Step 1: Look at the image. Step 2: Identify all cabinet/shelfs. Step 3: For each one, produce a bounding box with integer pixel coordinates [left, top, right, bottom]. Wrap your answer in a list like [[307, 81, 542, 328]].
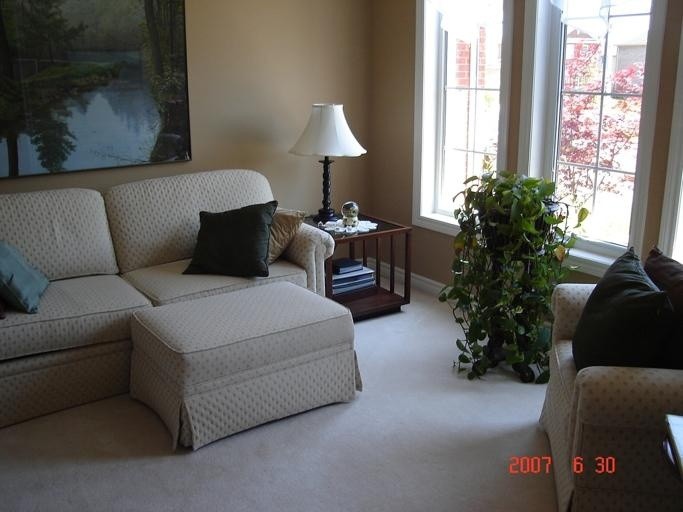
[[304, 211, 412, 321]]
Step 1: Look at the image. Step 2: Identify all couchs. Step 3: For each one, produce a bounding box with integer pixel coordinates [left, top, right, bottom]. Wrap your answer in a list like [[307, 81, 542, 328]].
[[1, 170, 335, 424]]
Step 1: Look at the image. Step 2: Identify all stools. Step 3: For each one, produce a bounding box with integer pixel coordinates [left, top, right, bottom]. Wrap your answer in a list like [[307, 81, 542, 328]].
[[129, 279, 362, 451]]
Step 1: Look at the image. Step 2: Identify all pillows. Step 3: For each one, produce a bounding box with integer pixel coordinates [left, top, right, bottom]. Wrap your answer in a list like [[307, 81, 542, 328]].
[[1, 239, 51, 313], [181, 201, 306, 277], [571, 245, 683, 372]]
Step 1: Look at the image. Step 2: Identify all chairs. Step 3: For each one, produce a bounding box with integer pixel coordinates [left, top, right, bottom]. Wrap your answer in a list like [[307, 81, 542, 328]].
[[537, 283, 682, 511]]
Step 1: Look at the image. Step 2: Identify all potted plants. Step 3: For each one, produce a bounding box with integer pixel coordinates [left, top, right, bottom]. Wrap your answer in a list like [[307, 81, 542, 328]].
[[438, 169, 588, 383]]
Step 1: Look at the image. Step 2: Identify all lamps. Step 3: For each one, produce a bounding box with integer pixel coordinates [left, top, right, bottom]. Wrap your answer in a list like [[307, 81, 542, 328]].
[[288, 103, 367, 224]]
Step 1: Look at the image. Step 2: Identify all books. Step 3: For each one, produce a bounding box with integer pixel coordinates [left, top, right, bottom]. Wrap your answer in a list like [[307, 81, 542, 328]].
[[328, 257, 378, 297]]
